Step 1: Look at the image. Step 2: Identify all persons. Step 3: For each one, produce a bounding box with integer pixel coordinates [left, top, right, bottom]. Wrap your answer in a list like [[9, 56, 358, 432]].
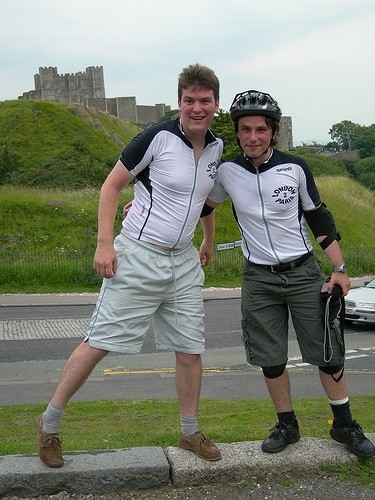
[[36, 62, 224, 468], [123, 90, 375, 459]]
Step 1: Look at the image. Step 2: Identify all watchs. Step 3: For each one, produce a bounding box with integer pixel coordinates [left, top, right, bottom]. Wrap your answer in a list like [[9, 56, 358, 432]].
[[333, 263, 348, 273]]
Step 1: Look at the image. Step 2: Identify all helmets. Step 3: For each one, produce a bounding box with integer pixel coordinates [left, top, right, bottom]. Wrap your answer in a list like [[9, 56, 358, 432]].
[[230, 90, 282, 122]]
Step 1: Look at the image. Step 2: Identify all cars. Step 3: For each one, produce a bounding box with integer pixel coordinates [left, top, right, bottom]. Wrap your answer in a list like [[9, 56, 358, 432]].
[[344, 277, 375, 328]]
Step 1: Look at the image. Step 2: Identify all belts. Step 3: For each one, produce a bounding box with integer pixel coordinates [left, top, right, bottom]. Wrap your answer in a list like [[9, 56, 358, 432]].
[[243, 250, 314, 273]]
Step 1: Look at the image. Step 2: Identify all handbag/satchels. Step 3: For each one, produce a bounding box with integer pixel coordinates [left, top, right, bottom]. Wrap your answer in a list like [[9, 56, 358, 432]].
[[320, 277, 346, 382]]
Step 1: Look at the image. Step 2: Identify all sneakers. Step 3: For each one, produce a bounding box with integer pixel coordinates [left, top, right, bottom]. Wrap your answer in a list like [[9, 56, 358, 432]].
[[261, 420, 300, 453], [179, 430, 221, 461], [36, 414, 64, 468], [330, 419, 375, 459]]
